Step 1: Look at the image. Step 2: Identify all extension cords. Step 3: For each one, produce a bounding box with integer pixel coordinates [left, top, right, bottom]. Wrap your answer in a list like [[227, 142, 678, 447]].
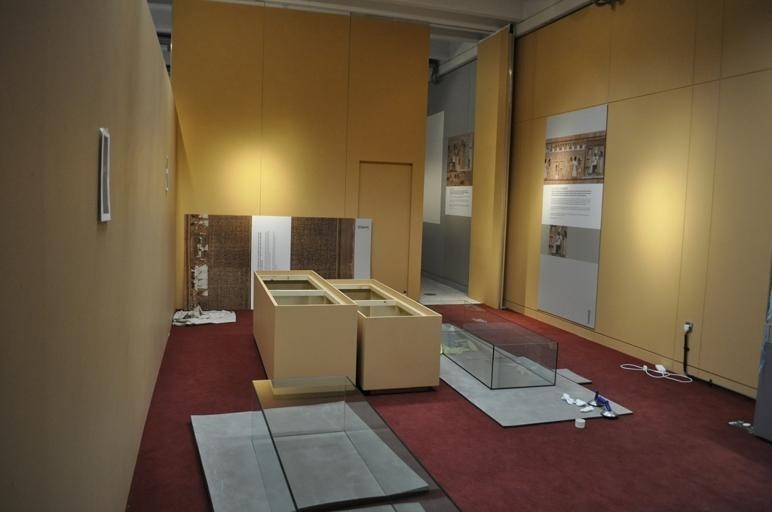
[[656, 364, 666, 373]]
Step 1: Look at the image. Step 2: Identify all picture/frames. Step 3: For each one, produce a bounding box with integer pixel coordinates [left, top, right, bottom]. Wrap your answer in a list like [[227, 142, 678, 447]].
[[99, 127, 112, 222]]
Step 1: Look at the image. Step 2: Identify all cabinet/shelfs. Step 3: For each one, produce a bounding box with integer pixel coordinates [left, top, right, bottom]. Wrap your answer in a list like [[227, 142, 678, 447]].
[[252, 269, 443, 399]]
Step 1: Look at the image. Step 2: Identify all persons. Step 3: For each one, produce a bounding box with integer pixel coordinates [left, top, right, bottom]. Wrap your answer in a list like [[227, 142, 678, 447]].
[[545, 150, 603, 179], [552, 231, 563, 254]]
